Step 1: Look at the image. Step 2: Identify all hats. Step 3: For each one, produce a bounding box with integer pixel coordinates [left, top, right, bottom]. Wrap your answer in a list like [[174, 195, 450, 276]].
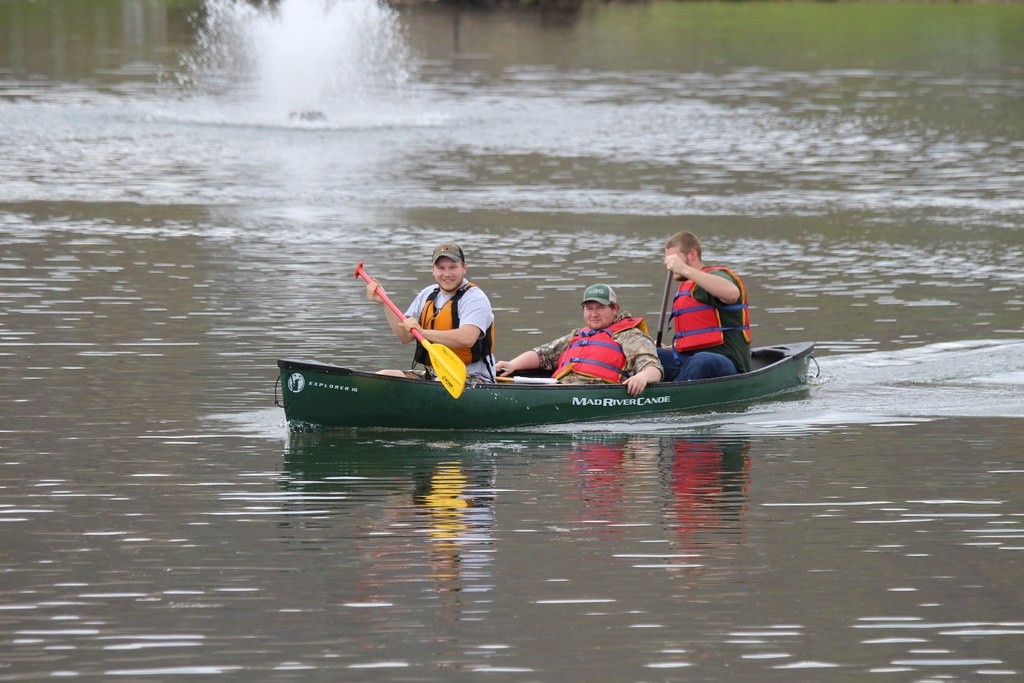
[[432, 242, 464, 265], [580, 283, 617, 305]]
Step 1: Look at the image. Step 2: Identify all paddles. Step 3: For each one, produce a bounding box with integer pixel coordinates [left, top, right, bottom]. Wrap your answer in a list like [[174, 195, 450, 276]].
[[656, 269, 674, 347], [355, 260, 467, 400]]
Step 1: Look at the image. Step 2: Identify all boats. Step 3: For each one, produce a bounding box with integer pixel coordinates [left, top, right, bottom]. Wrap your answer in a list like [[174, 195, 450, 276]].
[[275, 342, 816, 430]]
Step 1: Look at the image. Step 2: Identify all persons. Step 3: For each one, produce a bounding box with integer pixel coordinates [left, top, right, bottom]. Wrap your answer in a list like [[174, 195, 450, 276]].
[[653, 231, 752, 381], [367, 242, 495, 383], [495, 282, 664, 397]]
